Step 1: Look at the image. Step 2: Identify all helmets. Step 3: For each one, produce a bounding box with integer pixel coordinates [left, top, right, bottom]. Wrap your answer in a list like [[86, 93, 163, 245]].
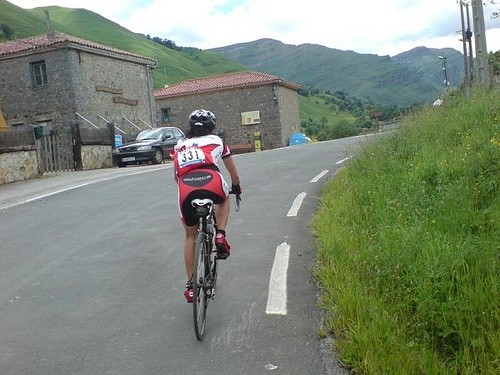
[[188, 108, 217, 127]]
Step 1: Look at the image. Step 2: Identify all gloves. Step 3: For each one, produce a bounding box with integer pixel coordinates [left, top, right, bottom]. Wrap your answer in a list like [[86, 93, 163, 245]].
[[231, 179, 242, 196]]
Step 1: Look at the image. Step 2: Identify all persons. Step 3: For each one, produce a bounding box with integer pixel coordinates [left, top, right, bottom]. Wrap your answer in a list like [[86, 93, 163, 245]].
[[171, 109, 243, 304]]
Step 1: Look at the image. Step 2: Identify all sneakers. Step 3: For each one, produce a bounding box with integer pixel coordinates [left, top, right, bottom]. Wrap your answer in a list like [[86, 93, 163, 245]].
[[185, 287, 197, 303], [215, 232, 231, 258]]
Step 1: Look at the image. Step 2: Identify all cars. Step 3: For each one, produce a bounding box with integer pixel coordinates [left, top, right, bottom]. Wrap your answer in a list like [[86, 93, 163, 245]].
[[112, 127, 186, 168]]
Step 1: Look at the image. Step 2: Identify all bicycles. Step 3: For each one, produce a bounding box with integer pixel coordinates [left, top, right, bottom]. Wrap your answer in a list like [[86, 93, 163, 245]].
[[186, 190, 240, 342]]
